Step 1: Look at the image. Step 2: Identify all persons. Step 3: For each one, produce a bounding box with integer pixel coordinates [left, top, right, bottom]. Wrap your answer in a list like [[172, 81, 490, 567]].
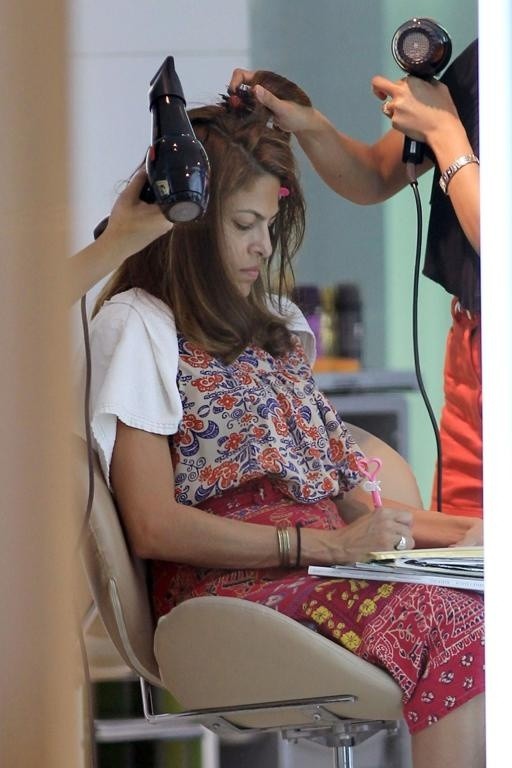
[[88, 98, 485, 768], [67, 165, 174, 310], [229, 38, 483, 519]]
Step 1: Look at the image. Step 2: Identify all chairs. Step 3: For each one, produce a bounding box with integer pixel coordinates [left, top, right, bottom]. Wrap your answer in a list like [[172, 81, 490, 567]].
[[76, 438, 407, 767]]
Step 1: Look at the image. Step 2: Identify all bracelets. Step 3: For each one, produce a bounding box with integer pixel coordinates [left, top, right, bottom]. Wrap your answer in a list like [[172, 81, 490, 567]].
[[438, 155, 478, 195], [276, 524, 302, 569]]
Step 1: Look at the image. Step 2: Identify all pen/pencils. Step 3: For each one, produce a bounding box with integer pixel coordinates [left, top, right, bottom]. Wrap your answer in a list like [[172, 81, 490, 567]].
[[357, 458, 382, 508]]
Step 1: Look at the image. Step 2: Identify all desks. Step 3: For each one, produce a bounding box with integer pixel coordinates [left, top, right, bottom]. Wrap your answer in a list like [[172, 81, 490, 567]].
[[318, 370, 419, 466]]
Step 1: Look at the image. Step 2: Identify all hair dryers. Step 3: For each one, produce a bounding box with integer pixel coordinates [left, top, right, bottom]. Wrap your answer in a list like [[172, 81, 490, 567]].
[[389, 18, 454, 167], [94, 56, 211, 241]]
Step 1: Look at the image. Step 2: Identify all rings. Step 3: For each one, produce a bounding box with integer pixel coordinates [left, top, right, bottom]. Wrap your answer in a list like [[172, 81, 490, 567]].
[[394, 535, 407, 551], [383, 102, 393, 115]]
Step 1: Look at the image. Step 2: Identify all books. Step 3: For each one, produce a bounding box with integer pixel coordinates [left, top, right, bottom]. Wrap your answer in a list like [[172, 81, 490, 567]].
[[307, 546, 484, 593]]
